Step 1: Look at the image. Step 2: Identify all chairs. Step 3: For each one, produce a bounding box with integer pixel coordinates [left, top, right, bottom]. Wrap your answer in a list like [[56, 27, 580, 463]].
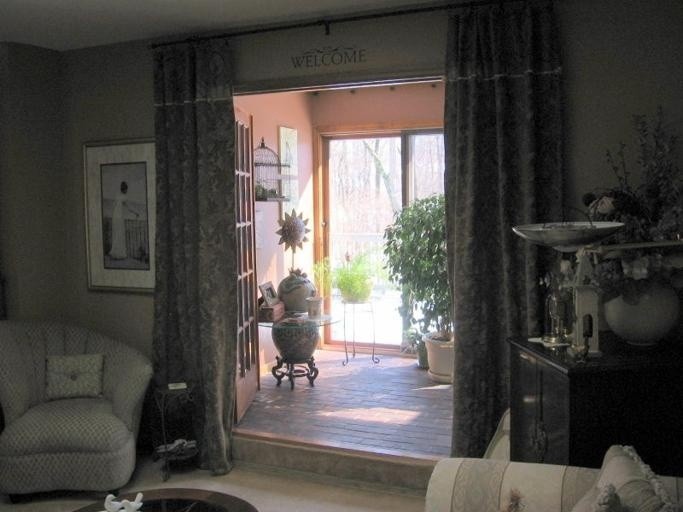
[[0, 320, 155, 504]]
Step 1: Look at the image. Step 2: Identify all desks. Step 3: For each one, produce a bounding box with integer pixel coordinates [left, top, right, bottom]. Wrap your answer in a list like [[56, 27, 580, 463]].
[[152, 382, 216, 481], [258, 312, 342, 390], [343, 299, 380, 365]]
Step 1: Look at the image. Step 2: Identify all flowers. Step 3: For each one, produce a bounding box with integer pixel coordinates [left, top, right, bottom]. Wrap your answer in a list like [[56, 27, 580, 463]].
[[571, 105, 683, 307]]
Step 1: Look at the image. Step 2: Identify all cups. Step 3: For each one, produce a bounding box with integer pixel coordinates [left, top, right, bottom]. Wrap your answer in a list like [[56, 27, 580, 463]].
[[304, 296, 324, 319]]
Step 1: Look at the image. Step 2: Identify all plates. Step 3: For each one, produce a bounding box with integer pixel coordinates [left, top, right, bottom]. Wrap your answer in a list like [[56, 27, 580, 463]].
[[509, 220, 625, 247]]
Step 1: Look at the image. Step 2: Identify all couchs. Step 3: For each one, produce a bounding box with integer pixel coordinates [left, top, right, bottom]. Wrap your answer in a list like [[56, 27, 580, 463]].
[[424, 458, 683, 512]]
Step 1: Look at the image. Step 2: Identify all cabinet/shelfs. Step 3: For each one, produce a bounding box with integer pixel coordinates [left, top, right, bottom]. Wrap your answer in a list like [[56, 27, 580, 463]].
[[506, 338, 683, 476]]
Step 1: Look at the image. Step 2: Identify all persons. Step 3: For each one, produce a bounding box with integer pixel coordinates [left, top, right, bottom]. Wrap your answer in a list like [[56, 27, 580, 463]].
[[108, 180, 140, 259]]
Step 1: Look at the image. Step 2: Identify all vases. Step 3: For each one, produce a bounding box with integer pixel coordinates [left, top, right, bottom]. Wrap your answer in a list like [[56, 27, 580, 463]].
[[604, 285, 681, 347]]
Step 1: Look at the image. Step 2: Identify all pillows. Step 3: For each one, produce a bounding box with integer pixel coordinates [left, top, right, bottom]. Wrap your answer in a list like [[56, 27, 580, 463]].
[[571, 445, 683, 512], [45, 353, 105, 401]]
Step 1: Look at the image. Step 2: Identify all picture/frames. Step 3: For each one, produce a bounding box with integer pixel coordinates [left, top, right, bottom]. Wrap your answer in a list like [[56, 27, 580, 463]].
[[259, 281, 280, 307], [82, 138, 158, 295]]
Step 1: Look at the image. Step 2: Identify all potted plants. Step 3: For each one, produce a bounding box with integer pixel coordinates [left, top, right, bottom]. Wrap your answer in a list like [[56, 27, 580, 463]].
[[400, 327, 430, 369], [312, 241, 377, 302], [307, 289, 325, 317], [384, 192, 454, 384]]
